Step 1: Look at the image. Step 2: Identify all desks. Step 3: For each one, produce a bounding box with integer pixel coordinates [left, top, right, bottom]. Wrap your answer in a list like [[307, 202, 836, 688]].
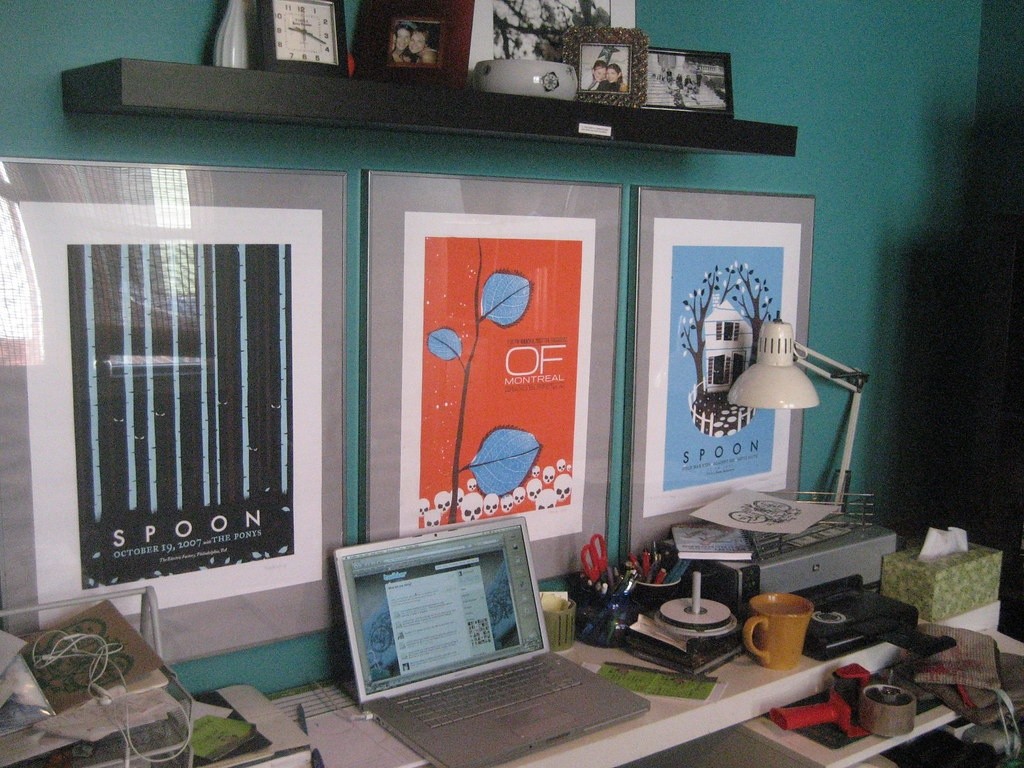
[[266, 600, 1024, 768]]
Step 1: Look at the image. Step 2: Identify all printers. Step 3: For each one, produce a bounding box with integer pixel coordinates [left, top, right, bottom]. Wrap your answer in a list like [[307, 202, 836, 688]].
[[656, 515, 957, 663]]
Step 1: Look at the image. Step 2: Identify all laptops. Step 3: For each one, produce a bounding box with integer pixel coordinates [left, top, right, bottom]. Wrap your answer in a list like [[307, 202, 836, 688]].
[[334, 516, 652, 768]]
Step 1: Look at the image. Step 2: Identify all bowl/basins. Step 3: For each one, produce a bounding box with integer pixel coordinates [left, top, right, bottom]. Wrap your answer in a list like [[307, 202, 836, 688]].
[[472, 60, 578, 101]]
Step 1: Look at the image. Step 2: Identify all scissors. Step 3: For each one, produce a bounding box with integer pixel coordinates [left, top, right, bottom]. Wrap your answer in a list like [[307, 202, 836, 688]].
[[581, 534, 608, 582]]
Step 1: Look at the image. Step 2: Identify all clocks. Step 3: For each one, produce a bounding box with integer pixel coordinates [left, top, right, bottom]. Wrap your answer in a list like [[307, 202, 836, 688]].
[[261, 0, 349, 80]]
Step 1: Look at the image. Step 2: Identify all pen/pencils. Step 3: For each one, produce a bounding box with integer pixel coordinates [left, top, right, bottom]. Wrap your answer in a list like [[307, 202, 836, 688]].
[[297, 703, 308, 735], [623, 541, 693, 585], [312, 748, 325, 768], [579, 566, 639, 648]]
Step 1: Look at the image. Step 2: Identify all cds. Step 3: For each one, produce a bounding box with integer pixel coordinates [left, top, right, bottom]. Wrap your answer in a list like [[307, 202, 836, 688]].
[[660, 597, 731, 625]]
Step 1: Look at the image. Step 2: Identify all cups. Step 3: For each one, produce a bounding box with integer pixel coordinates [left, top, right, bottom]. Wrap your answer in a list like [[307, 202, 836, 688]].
[[744, 592, 814, 671], [614, 563, 682, 623]]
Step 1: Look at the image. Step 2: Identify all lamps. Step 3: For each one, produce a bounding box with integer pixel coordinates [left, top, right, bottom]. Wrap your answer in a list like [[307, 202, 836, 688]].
[[728, 319, 870, 516]]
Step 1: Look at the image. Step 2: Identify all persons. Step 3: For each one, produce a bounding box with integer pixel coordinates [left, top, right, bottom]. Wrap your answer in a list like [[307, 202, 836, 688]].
[[581, 60, 628, 93], [660, 68, 703, 107], [392, 25, 438, 65]]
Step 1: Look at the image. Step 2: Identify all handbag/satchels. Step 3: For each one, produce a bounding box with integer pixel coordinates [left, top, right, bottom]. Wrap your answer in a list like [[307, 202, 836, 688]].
[[913, 624, 1024, 761]]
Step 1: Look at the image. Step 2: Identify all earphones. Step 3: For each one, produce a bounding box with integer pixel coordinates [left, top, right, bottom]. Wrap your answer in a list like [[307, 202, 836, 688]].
[[91, 681, 113, 706]]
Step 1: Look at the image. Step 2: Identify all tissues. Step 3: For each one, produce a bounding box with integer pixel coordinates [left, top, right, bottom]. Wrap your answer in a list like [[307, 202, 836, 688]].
[[880, 528, 1003, 625]]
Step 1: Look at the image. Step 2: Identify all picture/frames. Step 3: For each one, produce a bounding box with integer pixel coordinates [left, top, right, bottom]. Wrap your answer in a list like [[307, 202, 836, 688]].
[[0, 154, 345, 657], [616, 181, 818, 565], [466, 0, 636, 90], [386, 16, 446, 72], [361, 165, 623, 586], [561, 24, 648, 109], [640, 45, 735, 119]]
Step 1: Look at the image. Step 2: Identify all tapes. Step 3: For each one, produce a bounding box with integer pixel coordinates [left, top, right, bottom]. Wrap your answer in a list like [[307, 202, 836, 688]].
[[857, 686, 916, 738]]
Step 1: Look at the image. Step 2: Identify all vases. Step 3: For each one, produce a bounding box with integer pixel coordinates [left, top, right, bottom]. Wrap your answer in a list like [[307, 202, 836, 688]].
[[212, 0, 264, 71]]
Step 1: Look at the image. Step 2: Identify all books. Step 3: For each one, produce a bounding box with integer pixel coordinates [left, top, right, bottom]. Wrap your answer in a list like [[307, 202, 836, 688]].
[[671, 523, 754, 560]]
